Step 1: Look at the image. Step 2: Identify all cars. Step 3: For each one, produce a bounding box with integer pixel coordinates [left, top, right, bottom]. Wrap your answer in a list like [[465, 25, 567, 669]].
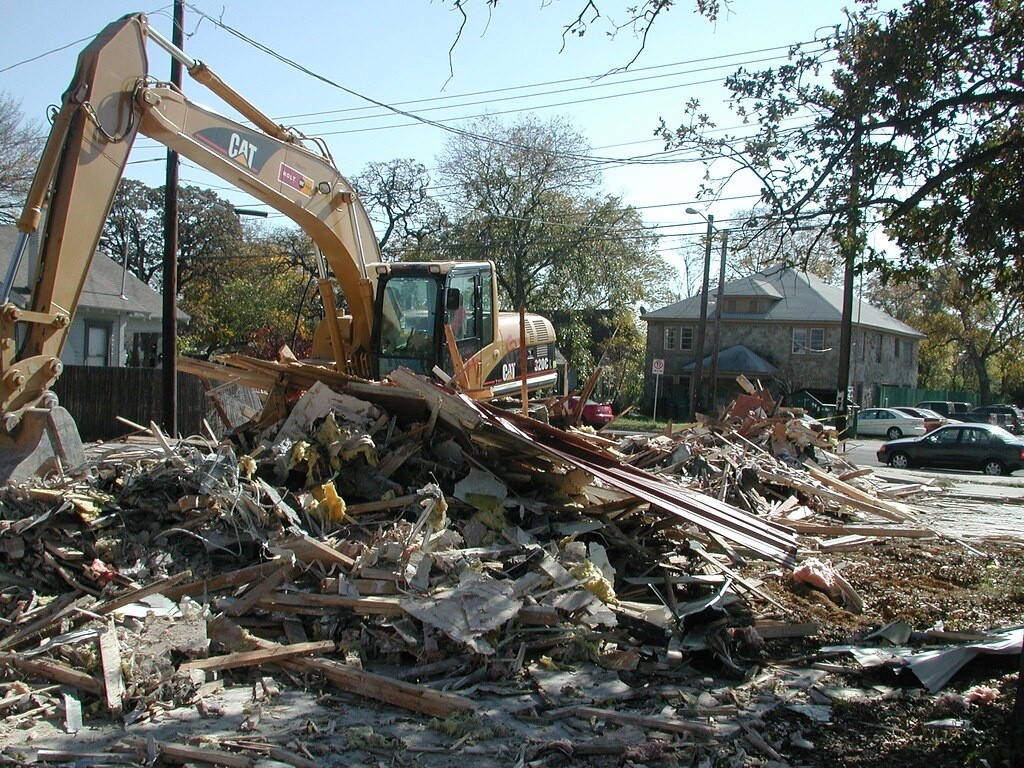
[[847, 408, 927, 439], [569, 395, 614, 428], [877, 422, 1024, 475], [888, 406, 942, 432]]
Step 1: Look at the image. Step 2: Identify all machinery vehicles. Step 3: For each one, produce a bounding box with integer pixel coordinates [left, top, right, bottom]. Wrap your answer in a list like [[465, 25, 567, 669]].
[[0, 7, 572, 491]]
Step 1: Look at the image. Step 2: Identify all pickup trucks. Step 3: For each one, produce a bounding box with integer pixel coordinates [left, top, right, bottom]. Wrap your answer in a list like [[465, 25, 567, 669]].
[[915, 400, 1015, 432]]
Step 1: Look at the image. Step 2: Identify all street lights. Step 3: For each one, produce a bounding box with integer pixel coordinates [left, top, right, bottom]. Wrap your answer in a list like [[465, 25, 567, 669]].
[[686, 207, 730, 415]]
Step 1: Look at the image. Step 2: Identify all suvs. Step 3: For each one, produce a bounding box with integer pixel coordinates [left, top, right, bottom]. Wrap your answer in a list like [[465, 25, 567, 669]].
[[973, 405, 1024, 434]]
